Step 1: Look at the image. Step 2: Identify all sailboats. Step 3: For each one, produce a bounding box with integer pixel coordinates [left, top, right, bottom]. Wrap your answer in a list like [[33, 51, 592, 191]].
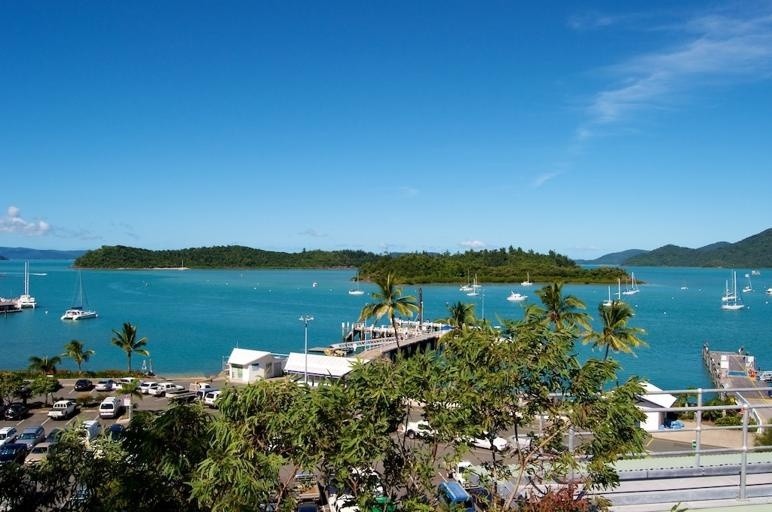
[[348, 272, 365, 296], [520, 271, 534, 286], [60, 269, 98, 321], [17, 259, 37, 310], [458, 269, 482, 297], [721, 267, 760, 311], [603, 271, 641, 309]]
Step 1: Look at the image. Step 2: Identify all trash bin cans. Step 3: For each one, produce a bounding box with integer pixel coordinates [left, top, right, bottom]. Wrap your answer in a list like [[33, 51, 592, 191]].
[[692, 440, 696, 448], [110, 424, 122, 440]]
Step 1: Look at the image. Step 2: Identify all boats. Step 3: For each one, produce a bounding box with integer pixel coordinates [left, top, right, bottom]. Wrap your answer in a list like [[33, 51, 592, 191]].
[[323, 347, 354, 358], [756, 370, 772, 381], [766, 287, 772, 295], [506, 290, 529, 301]]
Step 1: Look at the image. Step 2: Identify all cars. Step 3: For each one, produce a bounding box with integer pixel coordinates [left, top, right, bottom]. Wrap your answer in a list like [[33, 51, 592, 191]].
[[0, 376, 225, 468]]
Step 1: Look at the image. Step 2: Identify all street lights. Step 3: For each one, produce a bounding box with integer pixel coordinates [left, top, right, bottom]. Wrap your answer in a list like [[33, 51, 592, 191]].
[[299, 314, 315, 384], [420, 300, 424, 324], [339, 320, 389, 352]]
